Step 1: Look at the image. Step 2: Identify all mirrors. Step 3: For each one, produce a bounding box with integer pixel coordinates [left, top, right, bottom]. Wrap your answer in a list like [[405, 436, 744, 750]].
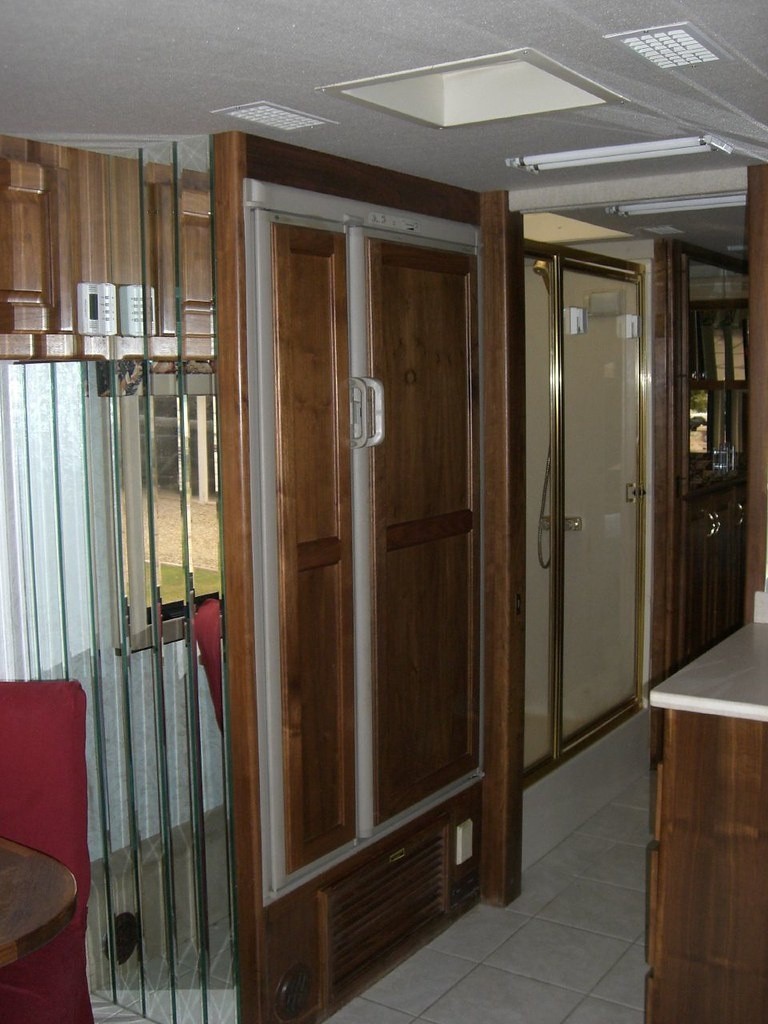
[[0, 134, 237, 1024]]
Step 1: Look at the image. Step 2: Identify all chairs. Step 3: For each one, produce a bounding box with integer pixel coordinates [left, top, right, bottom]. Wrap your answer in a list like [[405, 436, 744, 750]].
[[0, 679, 95, 1024], [195, 598, 222, 730]]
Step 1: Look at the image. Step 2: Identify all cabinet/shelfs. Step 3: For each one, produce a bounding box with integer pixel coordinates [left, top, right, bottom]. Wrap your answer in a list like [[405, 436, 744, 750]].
[[687, 482, 745, 664]]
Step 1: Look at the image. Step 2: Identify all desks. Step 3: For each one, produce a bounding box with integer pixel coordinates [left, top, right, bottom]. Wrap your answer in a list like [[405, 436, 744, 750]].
[[0, 836, 78, 967]]
